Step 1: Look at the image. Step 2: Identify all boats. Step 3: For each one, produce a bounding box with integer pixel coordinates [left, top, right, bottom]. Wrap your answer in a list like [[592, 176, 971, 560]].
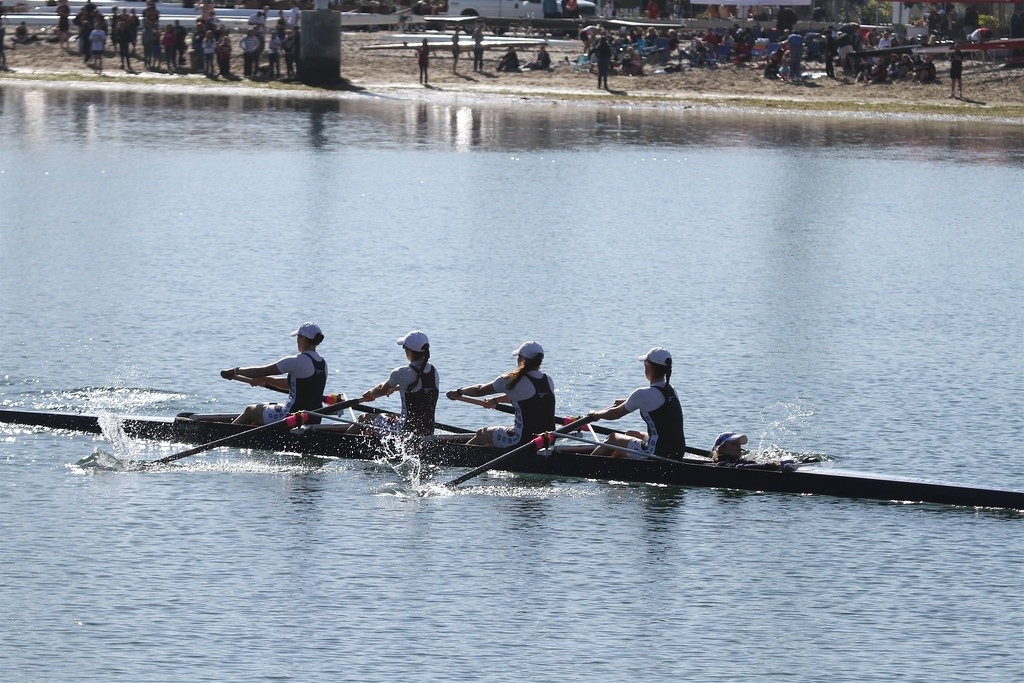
[[848, 37, 1024, 60], [0, 1, 428, 31], [425, 17, 687, 30], [359, 33, 569, 51], [0, 408, 1024, 510]]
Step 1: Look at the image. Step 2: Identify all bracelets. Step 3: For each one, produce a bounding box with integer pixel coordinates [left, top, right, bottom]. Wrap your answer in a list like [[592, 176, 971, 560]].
[[233, 367, 241, 374], [492, 397, 498, 405], [457, 386, 465, 397]]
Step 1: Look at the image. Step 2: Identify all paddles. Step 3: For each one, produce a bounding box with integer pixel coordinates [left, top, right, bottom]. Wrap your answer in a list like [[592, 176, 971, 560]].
[[445, 403, 618, 488], [447, 391, 714, 458], [153, 386, 399, 465], [220, 370, 478, 434]]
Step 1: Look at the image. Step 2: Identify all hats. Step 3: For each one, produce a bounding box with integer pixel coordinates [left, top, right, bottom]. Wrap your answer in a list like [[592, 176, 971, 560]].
[[712, 432, 747, 451], [397, 331, 429, 352], [290, 322, 322, 340], [512, 340, 544, 360], [639, 347, 672, 366]]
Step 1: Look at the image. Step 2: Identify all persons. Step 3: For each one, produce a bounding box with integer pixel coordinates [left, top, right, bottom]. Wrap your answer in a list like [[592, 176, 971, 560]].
[[559, 0, 1024, 99], [11, 21, 29, 43], [584, 346, 686, 461], [418, 38, 430, 85], [471, 28, 484, 73], [0, 25, 12, 72], [368, 0, 396, 13], [452, 25, 460, 74], [416, 0, 447, 15], [496, 45, 517, 72], [239, 0, 303, 83], [531, 46, 550, 71], [345, 329, 439, 441], [227, 322, 328, 424], [56, 0, 231, 78], [446, 341, 557, 446]]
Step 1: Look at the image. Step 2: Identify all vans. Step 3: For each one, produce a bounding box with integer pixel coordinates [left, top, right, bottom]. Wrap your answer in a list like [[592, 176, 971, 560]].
[[447, 0, 598, 39]]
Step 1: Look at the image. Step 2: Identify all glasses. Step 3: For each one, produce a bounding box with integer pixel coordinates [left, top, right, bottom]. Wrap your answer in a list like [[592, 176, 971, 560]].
[[403, 345, 409, 349]]
